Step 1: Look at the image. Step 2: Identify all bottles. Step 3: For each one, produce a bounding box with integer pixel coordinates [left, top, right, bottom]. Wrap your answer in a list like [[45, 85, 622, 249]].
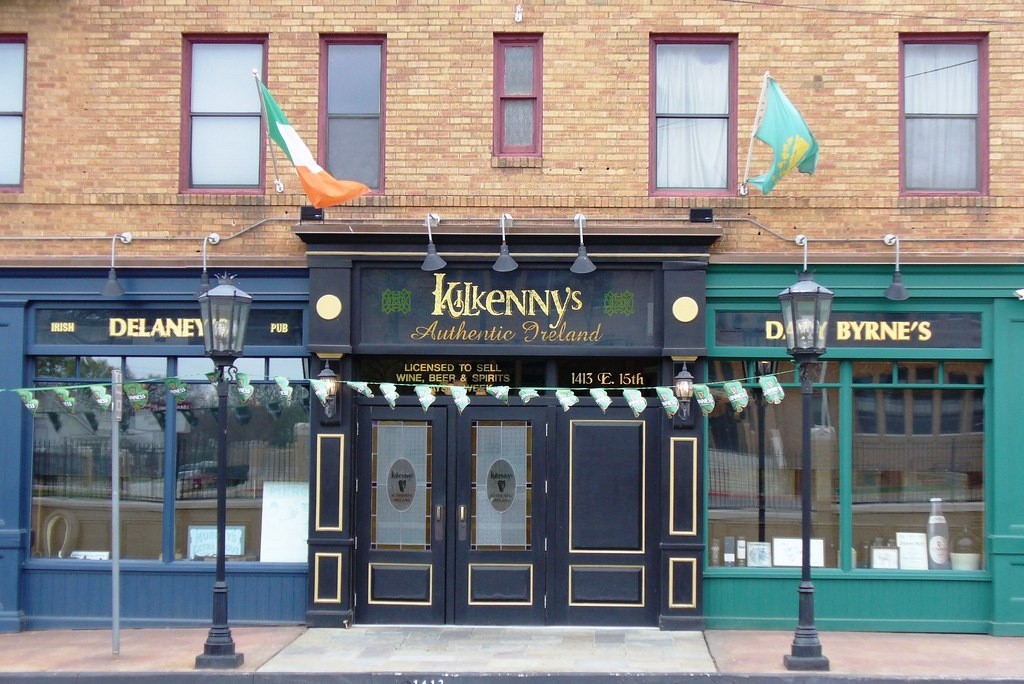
[[926, 498, 952, 570]]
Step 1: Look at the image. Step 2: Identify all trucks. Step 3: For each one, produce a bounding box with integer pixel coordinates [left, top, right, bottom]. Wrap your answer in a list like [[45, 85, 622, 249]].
[[181, 461, 248, 489]]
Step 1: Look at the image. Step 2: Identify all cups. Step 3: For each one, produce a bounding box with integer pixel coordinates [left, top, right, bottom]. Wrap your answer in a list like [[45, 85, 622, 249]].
[[949, 552, 981, 570]]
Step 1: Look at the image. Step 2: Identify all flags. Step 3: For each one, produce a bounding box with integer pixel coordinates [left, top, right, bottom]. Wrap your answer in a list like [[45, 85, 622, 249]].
[[746, 76, 819, 196], [259, 80, 371, 208]]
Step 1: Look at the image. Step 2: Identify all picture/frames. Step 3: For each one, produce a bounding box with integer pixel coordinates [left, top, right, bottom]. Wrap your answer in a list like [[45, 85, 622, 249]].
[[772, 536, 826, 567], [870, 546, 900, 570]]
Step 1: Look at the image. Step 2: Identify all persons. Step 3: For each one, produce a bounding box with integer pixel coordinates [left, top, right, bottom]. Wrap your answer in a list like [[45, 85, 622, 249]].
[[110, 440, 135, 496]]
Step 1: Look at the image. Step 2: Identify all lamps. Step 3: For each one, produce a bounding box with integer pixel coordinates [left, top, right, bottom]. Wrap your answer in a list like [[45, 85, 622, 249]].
[[100, 231, 133, 297], [491, 211, 518, 272], [420, 211, 447, 271], [194, 233, 220, 300], [882, 234, 909, 301], [570, 214, 597, 275], [792, 234, 827, 314], [673, 363, 694, 420], [317, 359, 338, 418]]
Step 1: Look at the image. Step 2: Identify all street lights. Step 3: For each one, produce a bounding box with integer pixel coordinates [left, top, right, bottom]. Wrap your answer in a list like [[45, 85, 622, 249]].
[[777, 270, 836, 672], [193, 270, 253, 669]]
